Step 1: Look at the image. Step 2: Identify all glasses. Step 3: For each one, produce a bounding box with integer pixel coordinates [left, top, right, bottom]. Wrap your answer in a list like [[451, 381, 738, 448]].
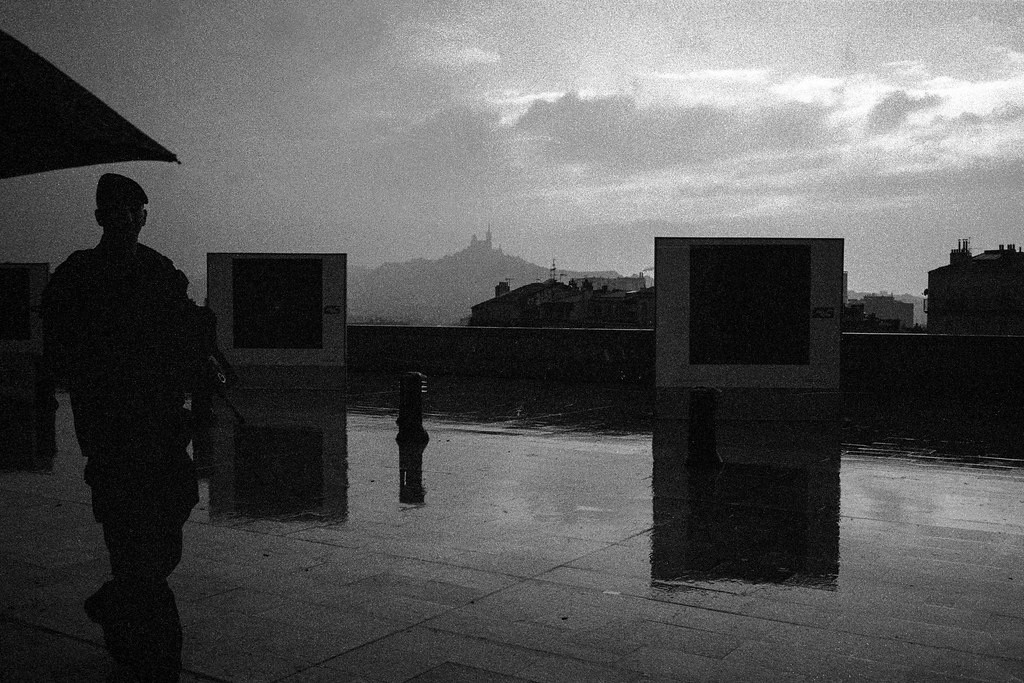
[[101, 201, 144, 220]]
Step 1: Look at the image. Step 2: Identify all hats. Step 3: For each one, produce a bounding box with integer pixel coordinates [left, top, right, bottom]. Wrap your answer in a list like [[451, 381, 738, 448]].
[[96, 173, 149, 205]]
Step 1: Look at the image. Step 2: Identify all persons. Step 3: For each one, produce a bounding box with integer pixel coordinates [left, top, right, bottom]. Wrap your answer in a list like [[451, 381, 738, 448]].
[[40, 174, 226, 683]]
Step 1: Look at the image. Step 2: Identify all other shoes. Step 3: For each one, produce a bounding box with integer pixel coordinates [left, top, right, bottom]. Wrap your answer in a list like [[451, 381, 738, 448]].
[[84, 582, 133, 660], [136, 618, 183, 683]]
